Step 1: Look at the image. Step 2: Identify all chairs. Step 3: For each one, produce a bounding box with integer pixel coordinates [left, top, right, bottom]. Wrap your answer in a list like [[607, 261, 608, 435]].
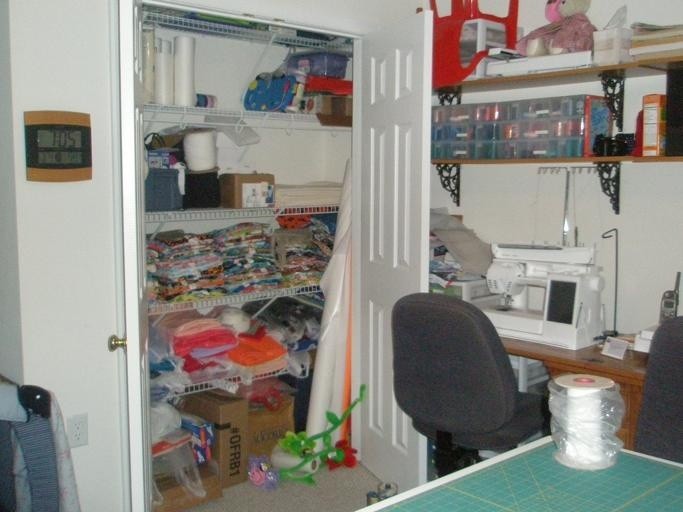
[[390, 297, 549, 478]]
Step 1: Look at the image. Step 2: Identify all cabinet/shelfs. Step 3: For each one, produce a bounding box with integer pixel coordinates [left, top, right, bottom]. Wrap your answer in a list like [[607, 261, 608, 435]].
[[434, 50, 682, 163], [135, 7, 343, 396]]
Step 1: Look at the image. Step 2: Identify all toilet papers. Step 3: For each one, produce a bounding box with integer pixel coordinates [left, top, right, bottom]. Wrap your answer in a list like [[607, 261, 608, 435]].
[[271, 453, 320, 473], [173, 34, 198, 108], [154, 52, 173, 106]]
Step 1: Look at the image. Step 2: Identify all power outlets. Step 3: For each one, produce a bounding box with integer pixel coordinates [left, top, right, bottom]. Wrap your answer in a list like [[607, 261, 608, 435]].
[[65, 413, 89, 449]]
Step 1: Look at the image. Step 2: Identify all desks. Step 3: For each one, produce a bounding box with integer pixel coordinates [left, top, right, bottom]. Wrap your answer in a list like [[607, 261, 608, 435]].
[[495, 331, 655, 454], [352, 431, 683, 509]]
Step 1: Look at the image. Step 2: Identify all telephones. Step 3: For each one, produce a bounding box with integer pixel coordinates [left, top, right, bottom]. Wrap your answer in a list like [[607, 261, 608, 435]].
[[658, 272, 682, 325]]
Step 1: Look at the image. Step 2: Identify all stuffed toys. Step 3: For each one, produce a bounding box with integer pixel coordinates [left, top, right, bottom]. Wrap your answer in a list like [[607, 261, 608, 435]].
[[514, 1, 594, 55]]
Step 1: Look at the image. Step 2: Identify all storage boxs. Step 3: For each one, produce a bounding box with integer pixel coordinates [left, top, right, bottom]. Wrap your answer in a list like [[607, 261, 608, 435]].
[[186, 381, 298, 490], [217, 172, 275, 208]]
